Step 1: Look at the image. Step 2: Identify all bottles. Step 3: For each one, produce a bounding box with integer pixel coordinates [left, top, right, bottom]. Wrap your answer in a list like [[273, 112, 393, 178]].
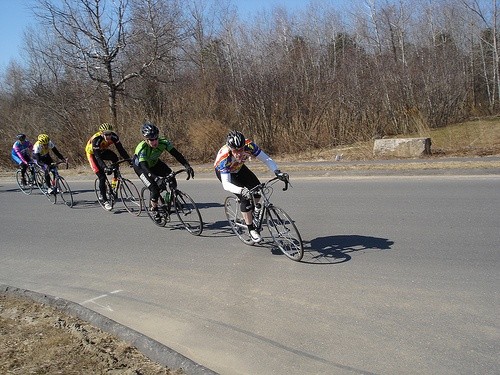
[[254, 202, 261, 218], [112, 177, 117, 189], [165, 191, 170, 203]]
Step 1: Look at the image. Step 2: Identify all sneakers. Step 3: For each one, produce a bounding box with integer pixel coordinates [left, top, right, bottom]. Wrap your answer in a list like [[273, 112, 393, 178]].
[[248, 230, 261, 243]]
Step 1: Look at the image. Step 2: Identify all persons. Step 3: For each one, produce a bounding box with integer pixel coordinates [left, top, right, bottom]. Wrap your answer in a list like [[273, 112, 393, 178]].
[[85, 122, 134, 210], [132, 124, 195, 211], [31, 134, 68, 195], [11, 134, 37, 188], [213, 130, 289, 243]]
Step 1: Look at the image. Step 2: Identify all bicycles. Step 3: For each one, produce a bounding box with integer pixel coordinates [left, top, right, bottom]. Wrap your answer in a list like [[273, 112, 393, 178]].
[[141, 168, 204, 236], [15, 161, 48, 195], [41, 156, 74, 208], [94, 157, 143, 216], [224, 172, 304, 262]]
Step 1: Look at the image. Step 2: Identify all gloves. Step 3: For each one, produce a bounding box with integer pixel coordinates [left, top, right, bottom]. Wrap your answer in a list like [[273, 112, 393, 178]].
[[275, 169, 288, 182], [240, 188, 251, 200], [103, 167, 112, 176], [129, 159, 134, 167], [185, 165, 194, 178], [156, 179, 167, 188]]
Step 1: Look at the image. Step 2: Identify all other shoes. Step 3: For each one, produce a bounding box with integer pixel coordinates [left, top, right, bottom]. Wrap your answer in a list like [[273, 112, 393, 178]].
[[177, 206, 192, 213], [21, 177, 26, 185], [47, 187, 53, 194], [151, 207, 161, 224], [104, 202, 112, 210]]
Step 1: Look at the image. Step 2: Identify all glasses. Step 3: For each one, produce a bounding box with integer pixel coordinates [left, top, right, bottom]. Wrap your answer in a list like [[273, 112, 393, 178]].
[[148, 136, 158, 142], [102, 132, 112, 136], [231, 148, 243, 153]]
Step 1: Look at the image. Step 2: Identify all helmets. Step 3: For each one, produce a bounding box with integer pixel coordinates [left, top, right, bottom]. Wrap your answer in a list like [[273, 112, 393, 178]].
[[98, 123, 112, 132], [37, 134, 50, 144], [226, 131, 245, 148], [16, 134, 26, 141], [142, 123, 160, 139]]
